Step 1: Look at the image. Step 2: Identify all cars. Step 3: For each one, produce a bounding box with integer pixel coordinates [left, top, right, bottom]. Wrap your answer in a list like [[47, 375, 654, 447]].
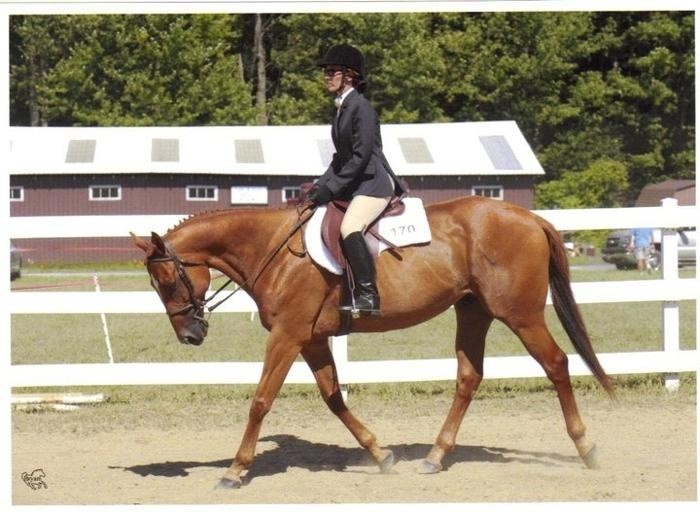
[[603, 227, 694, 272]]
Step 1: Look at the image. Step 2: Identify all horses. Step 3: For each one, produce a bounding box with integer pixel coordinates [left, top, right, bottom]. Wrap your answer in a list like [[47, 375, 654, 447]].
[[129, 193, 618, 493]]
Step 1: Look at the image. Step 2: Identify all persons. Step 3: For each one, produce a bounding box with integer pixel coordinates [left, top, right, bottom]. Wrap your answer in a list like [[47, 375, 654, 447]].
[[305, 44, 405, 317], [629, 229, 655, 278]]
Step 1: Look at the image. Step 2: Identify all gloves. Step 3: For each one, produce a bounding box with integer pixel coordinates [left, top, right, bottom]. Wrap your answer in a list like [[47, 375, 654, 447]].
[[305, 184, 333, 210]]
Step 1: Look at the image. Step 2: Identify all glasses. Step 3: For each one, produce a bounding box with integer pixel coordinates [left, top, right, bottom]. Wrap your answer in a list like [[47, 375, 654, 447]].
[[324, 69, 343, 76]]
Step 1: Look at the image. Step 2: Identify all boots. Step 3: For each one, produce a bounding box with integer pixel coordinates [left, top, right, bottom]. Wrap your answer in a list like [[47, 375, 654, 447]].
[[339, 232, 379, 319]]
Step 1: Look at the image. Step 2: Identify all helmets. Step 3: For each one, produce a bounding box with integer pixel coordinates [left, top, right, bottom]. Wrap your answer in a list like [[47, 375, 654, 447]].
[[316, 43, 365, 80]]
